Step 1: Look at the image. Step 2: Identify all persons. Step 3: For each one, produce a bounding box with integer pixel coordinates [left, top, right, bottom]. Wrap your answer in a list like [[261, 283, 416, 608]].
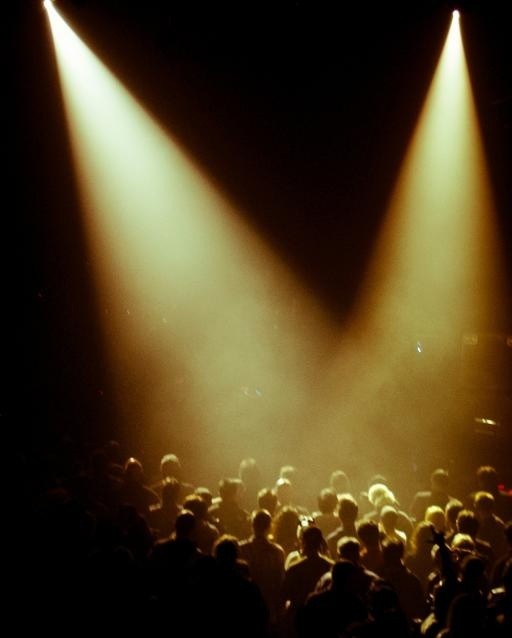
[[0, 437, 512, 638]]
